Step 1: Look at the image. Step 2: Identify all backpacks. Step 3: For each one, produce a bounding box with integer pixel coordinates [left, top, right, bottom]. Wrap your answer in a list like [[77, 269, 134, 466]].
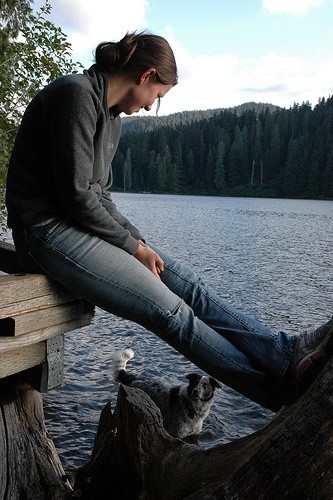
[[74, 440, 142, 500]]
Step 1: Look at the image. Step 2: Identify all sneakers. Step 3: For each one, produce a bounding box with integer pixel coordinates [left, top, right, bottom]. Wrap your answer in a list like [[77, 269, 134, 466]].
[[282, 316, 333, 408]]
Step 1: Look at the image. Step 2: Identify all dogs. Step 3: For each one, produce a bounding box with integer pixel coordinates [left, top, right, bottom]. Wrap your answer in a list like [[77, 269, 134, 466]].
[[109, 348, 223, 439]]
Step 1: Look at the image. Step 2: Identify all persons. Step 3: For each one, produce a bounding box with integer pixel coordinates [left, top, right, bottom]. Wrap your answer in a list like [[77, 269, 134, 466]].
[[4, 28, 333, 409]]
